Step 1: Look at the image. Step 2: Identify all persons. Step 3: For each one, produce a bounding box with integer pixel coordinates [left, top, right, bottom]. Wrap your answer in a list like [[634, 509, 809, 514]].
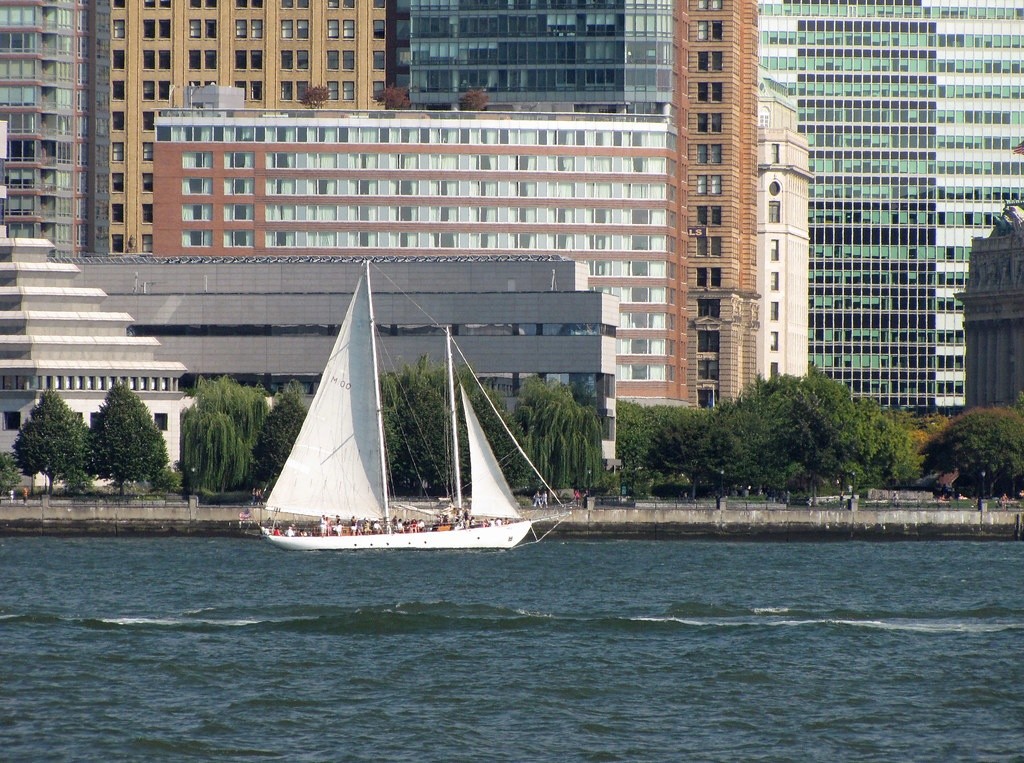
[[0, 490, 3, 504], [574, 489, 590, 509], [942, 494, 964, 500], [723, 486, 791, 507], [8, 488, 14, 504], [252, 487, 264, 505], [22, 486, 27, 506], [892, 491, 898, 507], [532, 490, 548, 509], [267, 509, 513, 537], [1002, 491, 1024, 501]]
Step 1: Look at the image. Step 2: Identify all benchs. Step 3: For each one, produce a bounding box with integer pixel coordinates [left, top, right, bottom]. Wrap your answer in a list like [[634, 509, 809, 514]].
[[996, 501, 1023, 512], [438, 497, 472, 505], [865, 500, 951, 508]]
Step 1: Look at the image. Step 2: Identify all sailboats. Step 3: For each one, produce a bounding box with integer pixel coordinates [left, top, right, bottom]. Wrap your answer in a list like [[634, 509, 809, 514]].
[[247, 260, 572, 552]]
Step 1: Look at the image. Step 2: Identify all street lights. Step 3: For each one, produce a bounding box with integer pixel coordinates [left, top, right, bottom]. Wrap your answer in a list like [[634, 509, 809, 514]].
[[43, 463, 48, 494], [190, 463, 197, 495], [587, 467, 592, 497], [851, 470, 855, 498], [720, 468, 725, 497], [982, 468, 986, 500]]
